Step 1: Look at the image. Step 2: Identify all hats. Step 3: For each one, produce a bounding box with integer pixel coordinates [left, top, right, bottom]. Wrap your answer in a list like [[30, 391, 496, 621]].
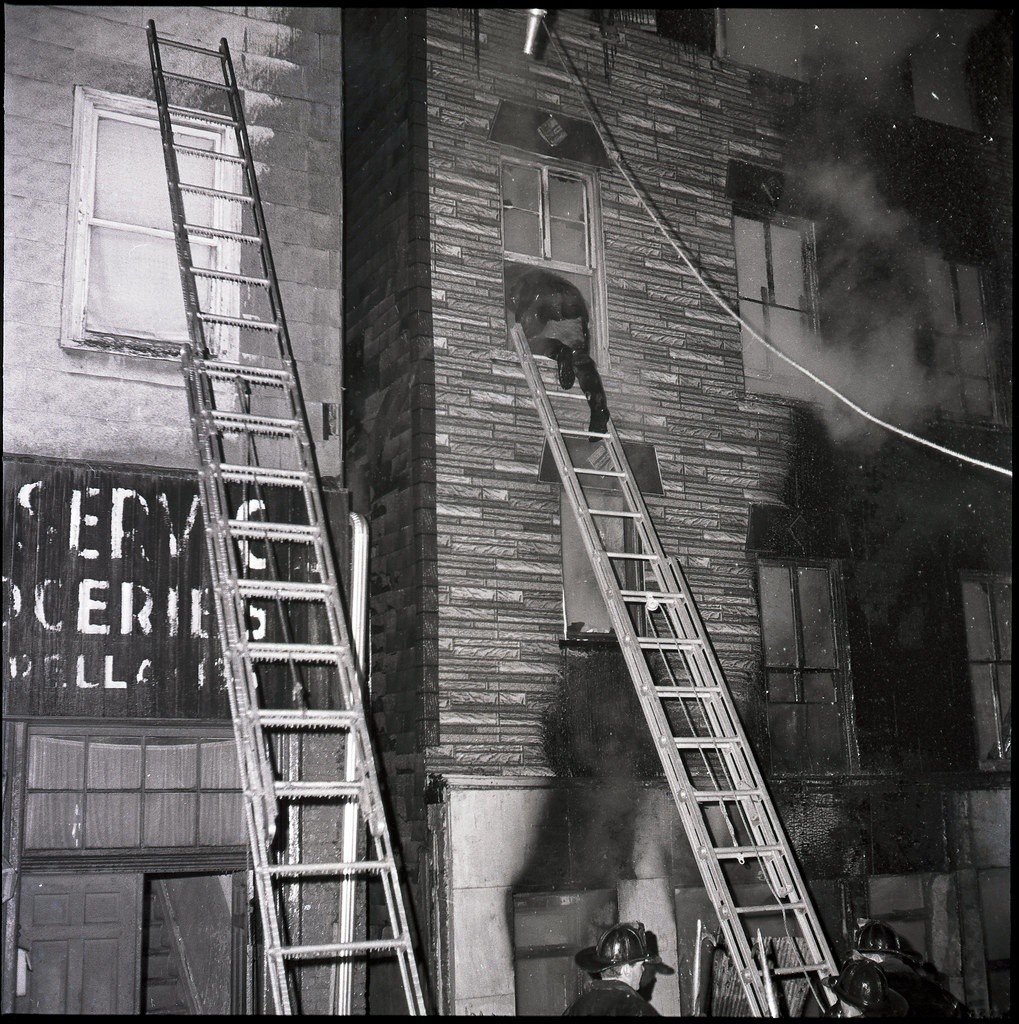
[[575, 923, 661, 972]]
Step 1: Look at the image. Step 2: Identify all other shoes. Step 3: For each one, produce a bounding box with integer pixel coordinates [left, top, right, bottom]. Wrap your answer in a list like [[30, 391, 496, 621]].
[[588, 407, 610, 443], [558, 346, 574, 390]]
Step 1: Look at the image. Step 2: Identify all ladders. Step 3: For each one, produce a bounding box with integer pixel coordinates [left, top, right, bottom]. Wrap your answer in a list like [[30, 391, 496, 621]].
[[138, 13, 432, 1024], [507, 321, 850, 1024]]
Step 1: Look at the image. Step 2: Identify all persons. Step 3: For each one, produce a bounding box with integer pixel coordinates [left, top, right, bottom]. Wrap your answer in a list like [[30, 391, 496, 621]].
[[508, 269, 610, 442], [563, 925, 667, 1021], [821, 920, 973, 1022]]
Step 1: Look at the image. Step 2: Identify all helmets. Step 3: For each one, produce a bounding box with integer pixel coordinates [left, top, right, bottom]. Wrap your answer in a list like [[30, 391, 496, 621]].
[[820, 959, 907, 1017], [852, 920, 914, 960]]
